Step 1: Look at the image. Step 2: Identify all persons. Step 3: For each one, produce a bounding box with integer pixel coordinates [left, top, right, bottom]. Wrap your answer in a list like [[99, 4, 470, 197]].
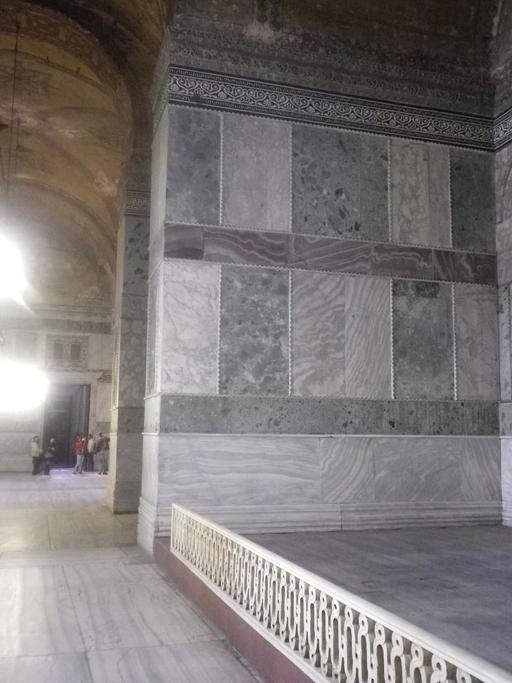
[[31, 436, 56, 475], [71, 432, 110, 474]]
